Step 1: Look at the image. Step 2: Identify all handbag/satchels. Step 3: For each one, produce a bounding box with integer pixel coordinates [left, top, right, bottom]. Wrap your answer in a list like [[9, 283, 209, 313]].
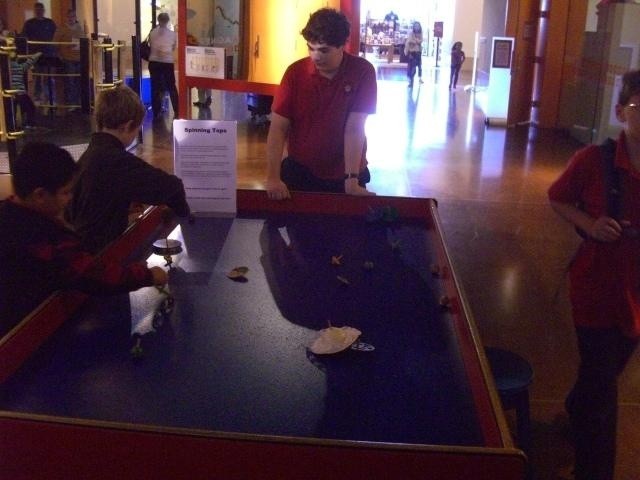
[[400, 54, 410, 63], [140, 37, 151, 59]]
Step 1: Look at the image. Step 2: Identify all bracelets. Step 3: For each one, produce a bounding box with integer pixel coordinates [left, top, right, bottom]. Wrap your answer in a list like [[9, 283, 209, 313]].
[[342, 170, 360, 180]]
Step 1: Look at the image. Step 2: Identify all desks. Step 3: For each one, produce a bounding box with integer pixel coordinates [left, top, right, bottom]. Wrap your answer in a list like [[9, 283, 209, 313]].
[[0, 186, 532, 479]]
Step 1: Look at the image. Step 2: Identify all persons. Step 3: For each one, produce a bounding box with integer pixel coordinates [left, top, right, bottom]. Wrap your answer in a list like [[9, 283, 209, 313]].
[[0, 140, 170, 343], [148, 12, 180, 120], [8, 51, 43, 132], [65, 86, 197, 259], [404, 21, 425, 86], [51, 8, 90, 103], [21, 2, 58, 101], [546, 71, 639, 479], [263, 9, 377, 201], [449, 41, 466, 90], [193, 87, 213, 107]]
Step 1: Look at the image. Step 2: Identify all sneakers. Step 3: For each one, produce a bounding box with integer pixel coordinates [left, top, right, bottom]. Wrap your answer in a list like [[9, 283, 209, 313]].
[[206, 96, 211, 104]]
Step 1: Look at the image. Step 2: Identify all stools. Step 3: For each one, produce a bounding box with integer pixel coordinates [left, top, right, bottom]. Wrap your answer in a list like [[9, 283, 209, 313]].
[[483, 346, 534, 452]]
